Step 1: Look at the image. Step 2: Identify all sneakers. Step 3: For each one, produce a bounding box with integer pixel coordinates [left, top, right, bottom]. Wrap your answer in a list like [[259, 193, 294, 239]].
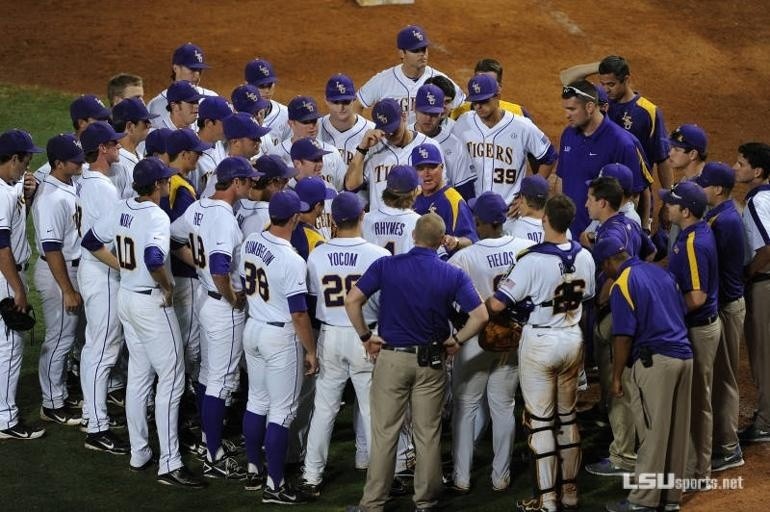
[[436, 453, 467, 491], [700, 433, 731, 469], [0, 423, 47, 442], [587, 454, 637, 477], [191, 415, 247, 478], [178, 408, 199, 454], [608, 496, 681, 511], [246, 464, 321, 506], [85, 382, 131, 456], [40, 390, 83, 425], [512, 489, 556, 511]]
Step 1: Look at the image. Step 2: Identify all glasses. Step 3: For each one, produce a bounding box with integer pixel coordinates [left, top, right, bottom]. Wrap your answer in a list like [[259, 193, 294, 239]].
[[561, 84, 598, 101]]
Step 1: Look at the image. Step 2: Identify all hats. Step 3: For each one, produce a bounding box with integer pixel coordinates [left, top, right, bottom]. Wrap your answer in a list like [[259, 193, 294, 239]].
[[327, 76, 500, 164], [288, 96, 322, 123], [244, 59, 278, 85], [144, 126, 301, 180], [167, 80, 272, 136], [0, 130, 45, 154], [469, 164, 708, 269], [395, 24, 432, 51], [269, 176, 367, 223], [69, 93, 161, 152], [173, 43, 210, 69], [134, 158, 181, 183], [387, 165, 425, 192], [659, 117, 718, 189], [47, 132, 85, 164]]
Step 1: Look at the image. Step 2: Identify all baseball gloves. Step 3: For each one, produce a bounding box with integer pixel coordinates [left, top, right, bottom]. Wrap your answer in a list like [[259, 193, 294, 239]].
[[1, 297, 36, 332], [479, 317, 522, 351]]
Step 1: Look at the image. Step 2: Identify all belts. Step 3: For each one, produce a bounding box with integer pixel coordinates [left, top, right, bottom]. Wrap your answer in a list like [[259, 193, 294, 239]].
[[266, 321, 286, 328], [207, 290, 223, 301], [40, 254, 80, 268], [380, 340, 449, 355], [13, 262, 30, 271], [685, 314, 718, 326], [135, 288, 153, 296]]
